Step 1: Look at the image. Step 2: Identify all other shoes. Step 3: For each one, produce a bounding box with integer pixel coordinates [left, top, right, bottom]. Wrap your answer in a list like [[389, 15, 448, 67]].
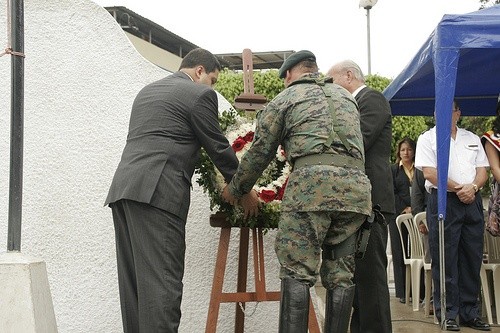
[[324, 284, 355, 333], [400, 298, 405, 304], [423, 304, 434, 315]]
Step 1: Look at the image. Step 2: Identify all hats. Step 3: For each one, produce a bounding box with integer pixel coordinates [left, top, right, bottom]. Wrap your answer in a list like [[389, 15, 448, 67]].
[[279, 50, 315, 79]]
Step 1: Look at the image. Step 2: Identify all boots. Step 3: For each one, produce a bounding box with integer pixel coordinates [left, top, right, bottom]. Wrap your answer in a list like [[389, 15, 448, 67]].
[[278, 278, 310, 333]]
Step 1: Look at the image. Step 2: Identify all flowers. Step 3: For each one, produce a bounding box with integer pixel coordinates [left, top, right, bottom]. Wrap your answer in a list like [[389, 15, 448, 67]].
[[191, 107, 293, 236]]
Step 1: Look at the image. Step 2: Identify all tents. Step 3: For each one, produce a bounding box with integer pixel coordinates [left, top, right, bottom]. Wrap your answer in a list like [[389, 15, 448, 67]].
[[379, 4, 500, 333]]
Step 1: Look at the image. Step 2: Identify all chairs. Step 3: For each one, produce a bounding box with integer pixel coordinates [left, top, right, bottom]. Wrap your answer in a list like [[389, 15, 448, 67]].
[[396, 213, 500, 327]]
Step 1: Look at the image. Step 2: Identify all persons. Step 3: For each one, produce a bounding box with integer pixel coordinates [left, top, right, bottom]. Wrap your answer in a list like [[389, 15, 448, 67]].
[[326, 61, 394, 333], [104, 48, 263, 333], [388, 139, 424, 303], [481, 95, 500, 235], [415, 97, 492, 331], [222, 50, 371, 333]]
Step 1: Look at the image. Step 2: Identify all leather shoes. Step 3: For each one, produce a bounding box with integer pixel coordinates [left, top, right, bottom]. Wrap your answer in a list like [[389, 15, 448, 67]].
[[440, 319, 460, 331], [459, 315, 490, 330]]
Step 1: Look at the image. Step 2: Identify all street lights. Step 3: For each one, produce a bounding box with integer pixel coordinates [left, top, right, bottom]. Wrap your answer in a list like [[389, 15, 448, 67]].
[[359, 0, 377, 77]]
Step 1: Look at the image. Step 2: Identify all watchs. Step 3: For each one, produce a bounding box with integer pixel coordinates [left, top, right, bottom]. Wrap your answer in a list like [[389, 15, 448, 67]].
[[471, 183, 478, 192]]
